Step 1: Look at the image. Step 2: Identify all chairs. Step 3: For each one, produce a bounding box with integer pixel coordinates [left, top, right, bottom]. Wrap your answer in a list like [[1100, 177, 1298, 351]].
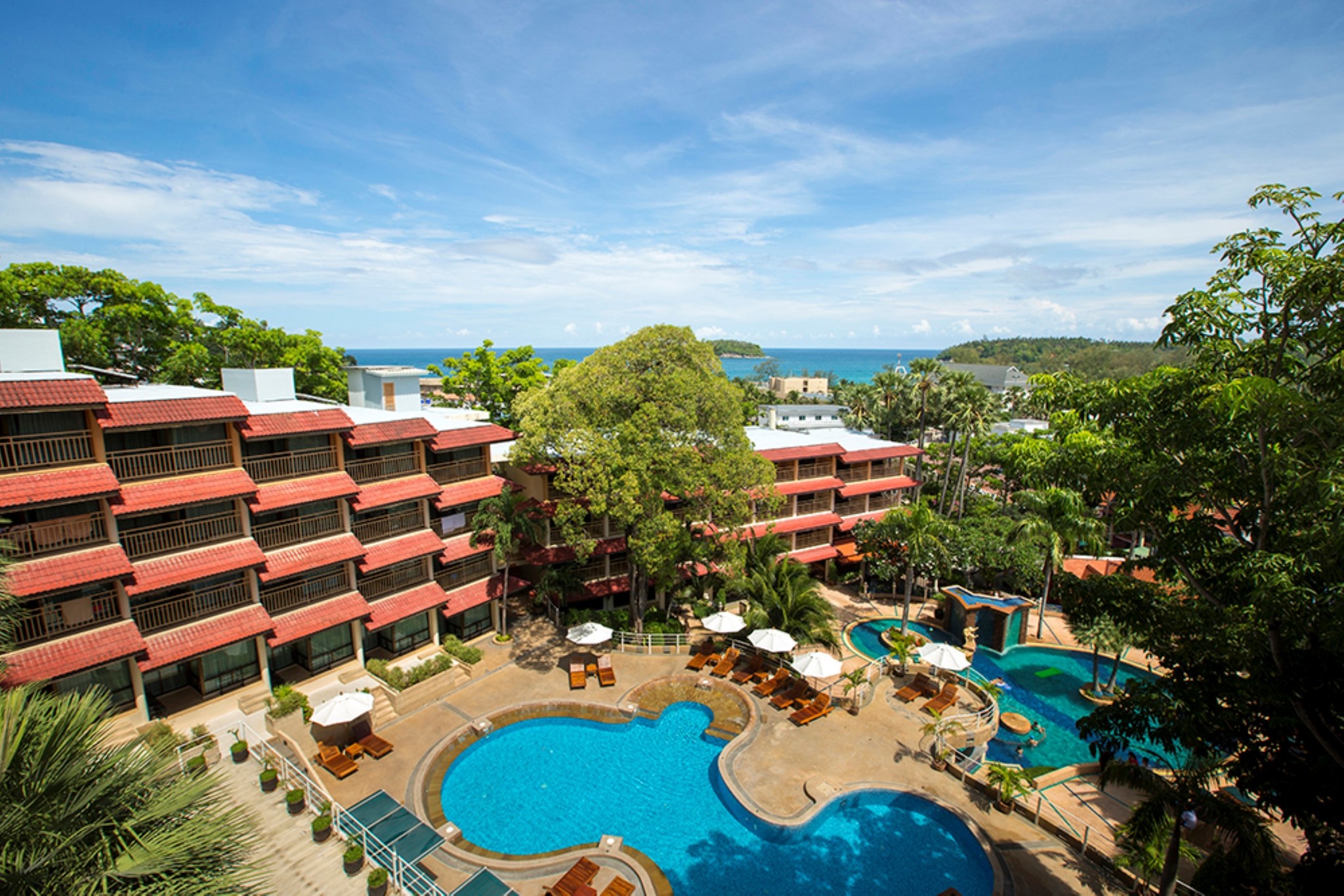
[[540, 855, 601, 896], [599, 875, 635, 896], [769, 677, 810, 710], [893, 671, 930, 703], [687, 641, 715, 671], [730, 654, 765, 685], [789, 691, 835, 727], [314, 735, 360, 780], [569, 655, 587, 690], [922, 682, 960, 717], [752, 667, 792, 698], [350, 719, 394, 761], [596, 652, 617, 687], [711, 647, 741, 677]]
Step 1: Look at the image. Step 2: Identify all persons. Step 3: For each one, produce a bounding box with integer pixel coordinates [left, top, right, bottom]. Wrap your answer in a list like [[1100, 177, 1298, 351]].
[[1034, 721, 1044, 733], [991, 677, 1004, 685], [1126, 751, 1139, 768], [1175, 806, 1201, 840], [1141, 756, 1153, 772], [1029, 735, 1036, 744], [1016, 745, 1024, 754]]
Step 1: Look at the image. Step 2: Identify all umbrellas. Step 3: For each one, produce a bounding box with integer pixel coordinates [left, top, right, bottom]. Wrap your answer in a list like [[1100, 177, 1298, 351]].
[[309, 691, 375, 744], [790, 650, 843, 700], [747, 627, 799, 668], [917, 639, 972, 688], [566, 622, 614, 665], [701, 611, 748, 655]]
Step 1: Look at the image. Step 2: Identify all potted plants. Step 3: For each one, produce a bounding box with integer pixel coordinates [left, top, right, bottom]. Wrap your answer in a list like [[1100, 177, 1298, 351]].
[[185, 744, 212, 781], [226, 726, 249, 763], [365, 654, 457, 713], [189, 723, 221, 766], [984, 760, 1033, 815], [840, 667, 874, 715], [262, 680, 311, 734], [367, 867, 389, 896], [311, 799, 332, 843], [442, 633, 486, 670], [342, 833, 366, 875], [916, 704, 968, 771], [284, 787, 306, 814], [258, 748, 279, 793]]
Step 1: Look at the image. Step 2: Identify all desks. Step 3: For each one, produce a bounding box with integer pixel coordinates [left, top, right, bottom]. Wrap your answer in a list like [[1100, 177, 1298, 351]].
[[708, 652, 722, 666], [572, 884, 597, 896], [585, 663, 597, 678], [925, 683, 938, 697], [344, 742, 364, 762], [794, 696, 812, 709], [754, 670, 768, 683]]
